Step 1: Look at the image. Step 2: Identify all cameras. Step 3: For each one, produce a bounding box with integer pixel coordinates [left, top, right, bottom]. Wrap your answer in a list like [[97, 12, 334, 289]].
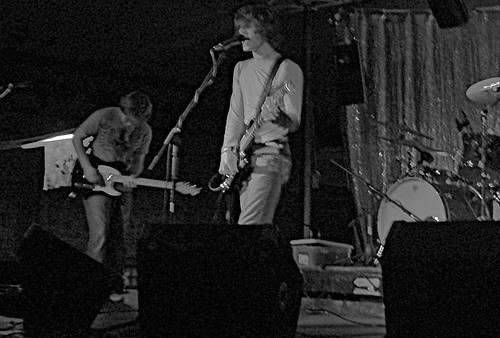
[[213, 34, 244, 51]]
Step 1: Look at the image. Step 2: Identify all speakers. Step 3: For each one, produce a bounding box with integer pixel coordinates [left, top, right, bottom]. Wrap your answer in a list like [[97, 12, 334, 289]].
[[380, 220, 500, 338], [136, 222, 304, 338], [16, 223, 122, 329]]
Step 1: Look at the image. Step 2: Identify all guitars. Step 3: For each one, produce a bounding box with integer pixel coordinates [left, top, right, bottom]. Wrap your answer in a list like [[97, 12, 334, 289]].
[[209, 80, 291, 191], [68, 154, 203, 199]]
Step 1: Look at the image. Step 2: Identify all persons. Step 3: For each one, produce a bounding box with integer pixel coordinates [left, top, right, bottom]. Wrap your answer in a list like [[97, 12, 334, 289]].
[[70, 89, 154, 304], [219, 5, 305, 224]]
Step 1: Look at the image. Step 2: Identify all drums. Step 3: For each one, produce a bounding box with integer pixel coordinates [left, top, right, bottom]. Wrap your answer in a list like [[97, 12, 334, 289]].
[[377, 177, 474, 247], [457, 135, 500, 189]]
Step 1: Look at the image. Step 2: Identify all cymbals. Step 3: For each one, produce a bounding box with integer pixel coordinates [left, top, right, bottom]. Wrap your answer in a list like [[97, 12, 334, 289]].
[[466, 77, 500, 105]]
